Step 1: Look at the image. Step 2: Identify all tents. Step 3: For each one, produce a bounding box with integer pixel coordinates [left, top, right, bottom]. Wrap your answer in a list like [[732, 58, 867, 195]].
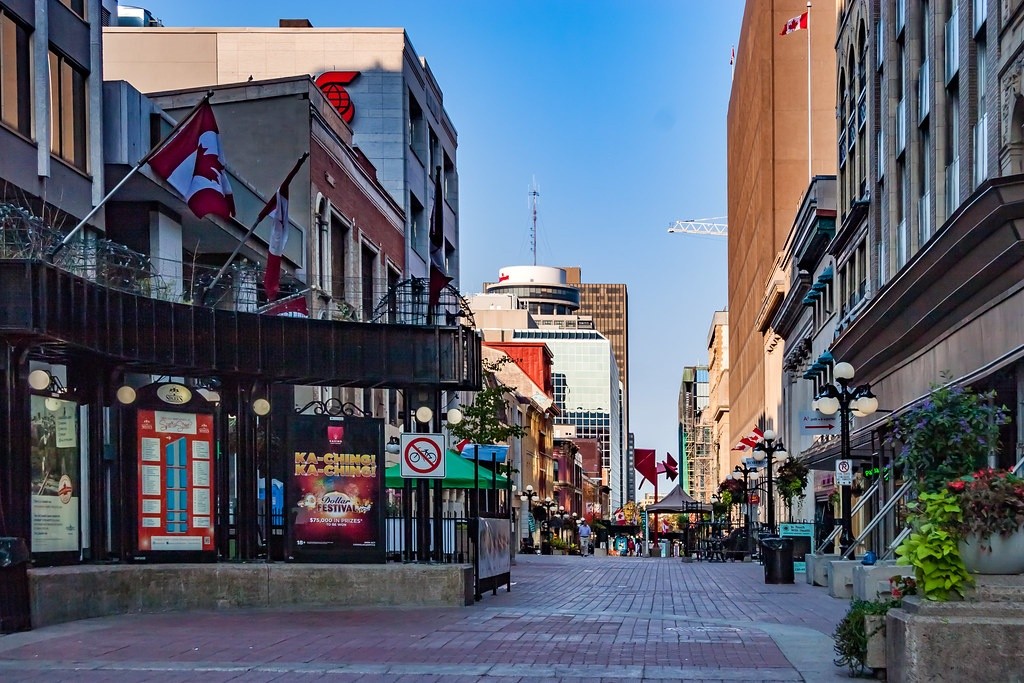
[[645, 484, 716, 556]]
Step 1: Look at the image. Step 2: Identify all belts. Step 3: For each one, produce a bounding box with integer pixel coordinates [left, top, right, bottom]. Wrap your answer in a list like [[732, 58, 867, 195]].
[[581, 536, 589, 538]]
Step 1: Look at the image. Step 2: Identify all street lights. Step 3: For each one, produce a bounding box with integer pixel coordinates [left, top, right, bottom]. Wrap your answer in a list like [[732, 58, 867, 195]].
[[751, 429, 788, 533], [519, 484, 540, 547], [732, 455, 762, 538], [555, 504, 570, 544], [811, 362, 880, 562], [568, 511, 583, 544], [538, 496, 558, 551]]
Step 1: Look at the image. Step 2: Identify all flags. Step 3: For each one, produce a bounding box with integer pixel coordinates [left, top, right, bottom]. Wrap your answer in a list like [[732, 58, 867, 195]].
[[729, 427, 764, 454], [262, 180, 290, 302], [662, 519, 670, 536], [615, 508, 627, 526], [147, 97, 237, 219]]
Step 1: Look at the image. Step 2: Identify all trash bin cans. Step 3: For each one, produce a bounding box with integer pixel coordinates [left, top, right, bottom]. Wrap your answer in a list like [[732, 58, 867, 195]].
[[762, 538, 795, 586], [542, 542, 551, 554]]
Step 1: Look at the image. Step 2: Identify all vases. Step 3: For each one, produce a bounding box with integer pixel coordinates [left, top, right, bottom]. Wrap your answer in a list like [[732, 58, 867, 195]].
[[953, 525, 1024, 575]]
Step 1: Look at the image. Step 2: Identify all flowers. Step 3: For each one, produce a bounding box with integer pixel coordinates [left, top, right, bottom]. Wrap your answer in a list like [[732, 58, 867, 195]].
[[941, 466, 1024, 552]]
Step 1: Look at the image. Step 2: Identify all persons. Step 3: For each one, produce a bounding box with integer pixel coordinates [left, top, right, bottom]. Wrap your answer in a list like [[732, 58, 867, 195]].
[[579, 520, 591, 558], [628, 538, 634, 556]]
